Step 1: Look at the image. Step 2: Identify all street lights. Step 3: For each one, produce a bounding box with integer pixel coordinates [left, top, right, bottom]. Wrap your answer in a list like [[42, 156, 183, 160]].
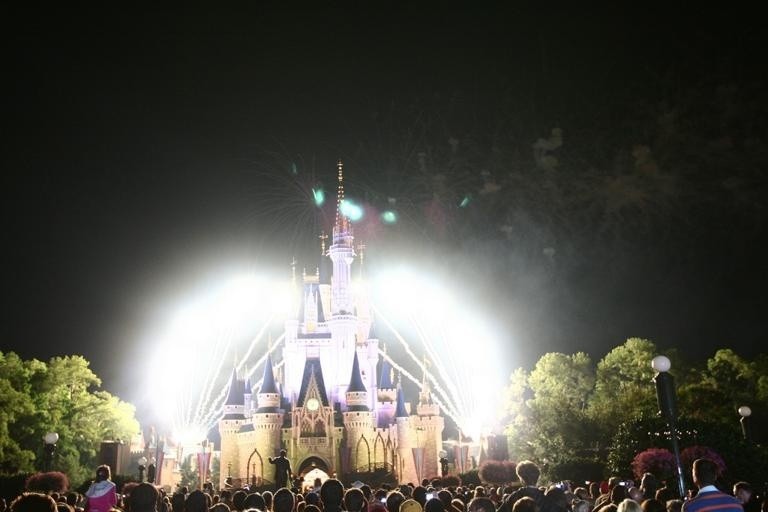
[[738, 406, 752, 441], [439, 450, 448, 477], [44, 432, 59, 471], [138, 456, 156, 483], [652, 354, 687, 499], [543, 458, 548, 477]]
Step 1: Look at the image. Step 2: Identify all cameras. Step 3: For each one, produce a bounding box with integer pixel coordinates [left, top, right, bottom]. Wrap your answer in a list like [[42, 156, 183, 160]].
[[425, 493, 438, 500], [619, 481, 629, 487], [555, 485, 561, 488], [380, 498, 385, 502]]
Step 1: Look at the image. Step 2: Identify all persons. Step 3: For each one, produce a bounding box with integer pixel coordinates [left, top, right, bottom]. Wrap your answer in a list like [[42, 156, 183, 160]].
[[1, 448, 768, 512]]
